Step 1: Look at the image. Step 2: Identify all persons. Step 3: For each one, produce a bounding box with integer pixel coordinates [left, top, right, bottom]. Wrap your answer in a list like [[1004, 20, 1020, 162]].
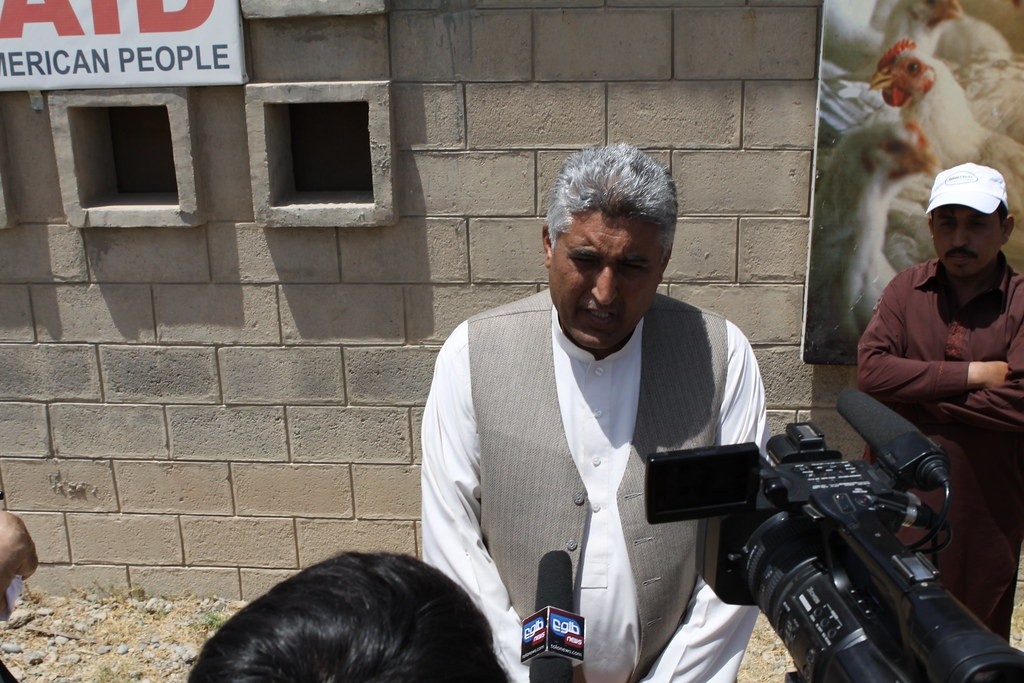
[[420, 142, 770, 683], [856, 161, 1024, 649], [0, 510, 40, 683], [186, 551, 517, 683]]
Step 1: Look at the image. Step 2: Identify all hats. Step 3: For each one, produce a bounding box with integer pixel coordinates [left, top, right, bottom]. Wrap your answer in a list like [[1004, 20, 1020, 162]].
[[925, 162, 1009, 214]]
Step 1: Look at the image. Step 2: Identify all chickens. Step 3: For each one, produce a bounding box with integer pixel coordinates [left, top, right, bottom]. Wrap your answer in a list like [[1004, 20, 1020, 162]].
[[807, 0, 1024, 361]]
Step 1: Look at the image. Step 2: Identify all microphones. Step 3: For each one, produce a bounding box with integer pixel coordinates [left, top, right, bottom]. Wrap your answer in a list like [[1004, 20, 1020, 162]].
[[836, 387, 951, 492], [521, 550, 584, 683]]
